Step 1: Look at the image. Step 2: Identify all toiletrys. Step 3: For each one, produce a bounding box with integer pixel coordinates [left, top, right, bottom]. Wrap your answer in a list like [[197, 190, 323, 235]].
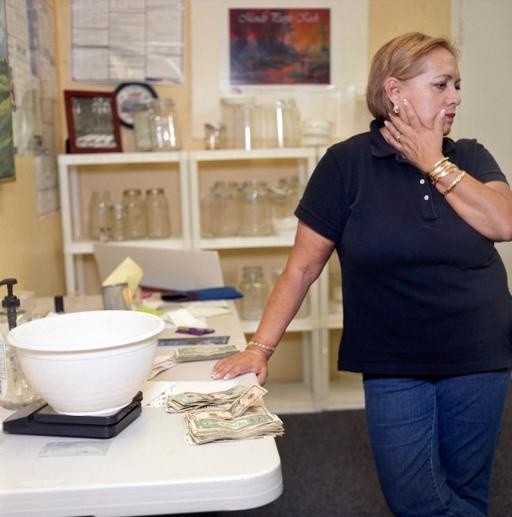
[[0, 277, 41, 411]]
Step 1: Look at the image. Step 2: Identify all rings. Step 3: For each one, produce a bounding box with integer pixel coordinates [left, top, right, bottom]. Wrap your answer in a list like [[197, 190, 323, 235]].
[[395, 132, 402, 143]]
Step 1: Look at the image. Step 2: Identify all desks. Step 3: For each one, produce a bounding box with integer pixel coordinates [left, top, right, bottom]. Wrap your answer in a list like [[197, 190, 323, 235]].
[[1, 296, 285, 517]]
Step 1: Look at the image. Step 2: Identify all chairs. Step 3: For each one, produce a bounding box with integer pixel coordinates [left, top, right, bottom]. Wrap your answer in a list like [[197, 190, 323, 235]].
[[90, 243, 227, 306]]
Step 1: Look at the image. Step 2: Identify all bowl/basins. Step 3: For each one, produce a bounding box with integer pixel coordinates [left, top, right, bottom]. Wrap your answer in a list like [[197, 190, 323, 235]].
[[7, 310, 166, 417]]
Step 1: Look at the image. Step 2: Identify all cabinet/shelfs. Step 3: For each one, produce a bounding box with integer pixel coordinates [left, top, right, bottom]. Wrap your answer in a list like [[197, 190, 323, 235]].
[[316, 149, 366, 413], [58, 153, 189, 298], [190, 149, 319, 410]]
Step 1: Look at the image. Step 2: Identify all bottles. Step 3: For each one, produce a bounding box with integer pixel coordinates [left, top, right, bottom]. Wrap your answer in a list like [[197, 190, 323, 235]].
[[86, 186, 171, 242], [237, 265, 311, 320], [206, 178, 305, 239], [129, 91, 302, 152]]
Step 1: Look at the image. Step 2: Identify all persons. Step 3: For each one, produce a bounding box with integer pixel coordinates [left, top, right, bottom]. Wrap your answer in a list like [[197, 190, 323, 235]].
[[209, 31, 512, 515]]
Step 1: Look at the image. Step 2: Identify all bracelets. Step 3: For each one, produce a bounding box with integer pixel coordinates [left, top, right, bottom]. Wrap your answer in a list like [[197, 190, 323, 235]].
[[247, 339, 278, 352], [424, 155, 459, 186], [440, 169, 467, 198]]
